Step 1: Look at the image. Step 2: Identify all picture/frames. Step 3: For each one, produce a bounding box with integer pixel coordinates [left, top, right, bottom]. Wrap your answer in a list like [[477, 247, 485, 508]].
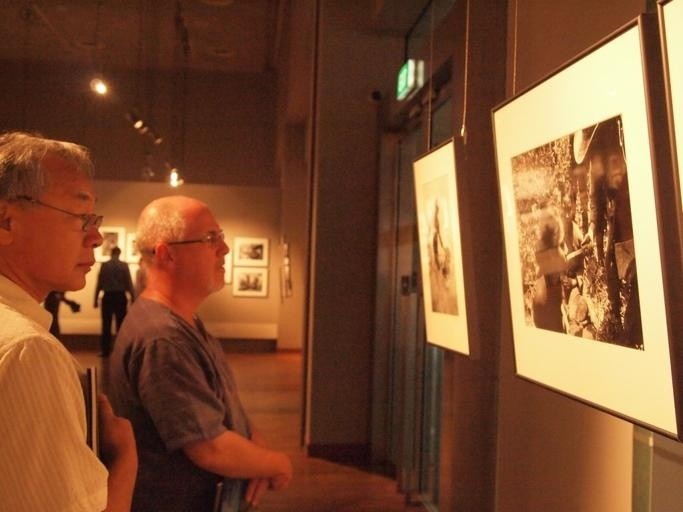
[[411, 134, 481, 361], [94, 225, 144, 300], [654, 0, 682, 256], [224, 234, 272, 298], [488, 10, 683, 443]]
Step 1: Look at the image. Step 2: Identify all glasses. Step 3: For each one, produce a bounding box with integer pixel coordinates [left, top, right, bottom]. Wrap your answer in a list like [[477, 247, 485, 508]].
[[150, 232, 224, 254], [12, 192, 104, 232]]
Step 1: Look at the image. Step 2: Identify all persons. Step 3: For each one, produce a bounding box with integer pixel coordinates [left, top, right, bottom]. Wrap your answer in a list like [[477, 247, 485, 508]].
[[0, 129, 140, 512], [423, 198, 459, 317], [526, 117, 641, 347], [93, 244, 137, 357], [45, 290, 80, 339], [96, 195, 295, 512]]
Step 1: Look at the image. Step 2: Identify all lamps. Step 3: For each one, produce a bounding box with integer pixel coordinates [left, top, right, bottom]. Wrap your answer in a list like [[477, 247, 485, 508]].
[[123, 106, 184, 187]]
